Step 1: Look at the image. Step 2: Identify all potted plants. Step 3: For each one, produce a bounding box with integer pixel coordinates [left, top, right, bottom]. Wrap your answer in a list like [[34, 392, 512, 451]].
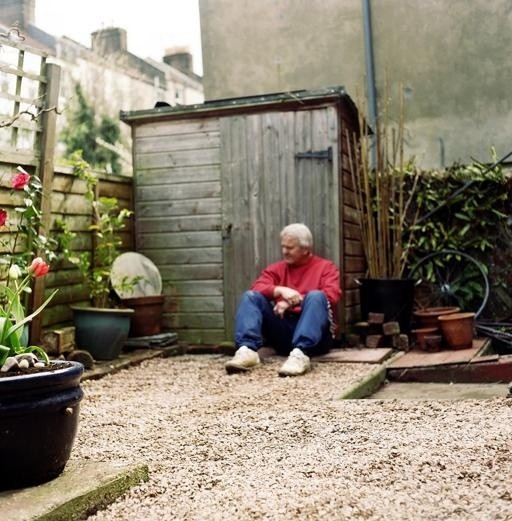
[[120, 275, 167, 337], [54, 154, 135, 361]]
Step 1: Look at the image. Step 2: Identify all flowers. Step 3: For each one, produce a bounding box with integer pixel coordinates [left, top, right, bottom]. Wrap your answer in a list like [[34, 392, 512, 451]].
[[0, 166, 60, 369]]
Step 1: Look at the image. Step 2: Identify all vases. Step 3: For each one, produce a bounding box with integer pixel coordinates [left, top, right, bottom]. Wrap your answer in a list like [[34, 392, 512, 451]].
[[411, 306, 476, 353], [0, 360, 84, 493]]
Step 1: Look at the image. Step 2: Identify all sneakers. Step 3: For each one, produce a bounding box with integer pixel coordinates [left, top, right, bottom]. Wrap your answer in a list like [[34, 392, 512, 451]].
[[278, 348, 311, 378], [226, 346, 262, 374]]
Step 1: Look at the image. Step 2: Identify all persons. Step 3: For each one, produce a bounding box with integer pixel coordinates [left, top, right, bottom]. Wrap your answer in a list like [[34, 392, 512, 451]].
[[226, 223, 342, 377]]
